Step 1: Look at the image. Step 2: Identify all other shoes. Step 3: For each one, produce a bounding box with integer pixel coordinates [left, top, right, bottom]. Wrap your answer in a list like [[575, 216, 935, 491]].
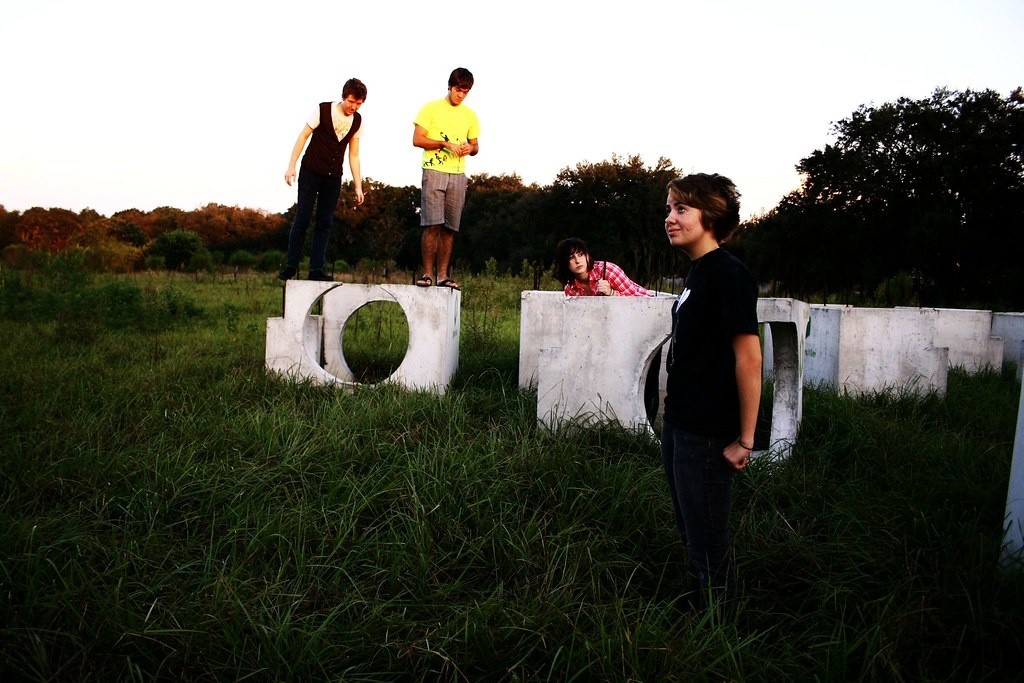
[[278, 266, 297, 280], [306, 270, 335, 282]]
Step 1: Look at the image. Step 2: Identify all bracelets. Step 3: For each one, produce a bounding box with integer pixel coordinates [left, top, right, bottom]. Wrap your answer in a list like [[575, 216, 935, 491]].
[[738, 437, 752, 451], [608, 289, 615, 296]]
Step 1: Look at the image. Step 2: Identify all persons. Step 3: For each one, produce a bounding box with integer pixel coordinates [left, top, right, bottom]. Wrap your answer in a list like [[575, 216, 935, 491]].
[[556, 238, 654, 297], [413, 68, 480, 290], [278, 78, 368, 281], [660, 172, 762, 593]]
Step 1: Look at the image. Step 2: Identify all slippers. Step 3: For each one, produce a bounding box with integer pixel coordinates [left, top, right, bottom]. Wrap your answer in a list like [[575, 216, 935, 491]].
[[437, 278, 461, 291], [417, 276, 434, 288]]
